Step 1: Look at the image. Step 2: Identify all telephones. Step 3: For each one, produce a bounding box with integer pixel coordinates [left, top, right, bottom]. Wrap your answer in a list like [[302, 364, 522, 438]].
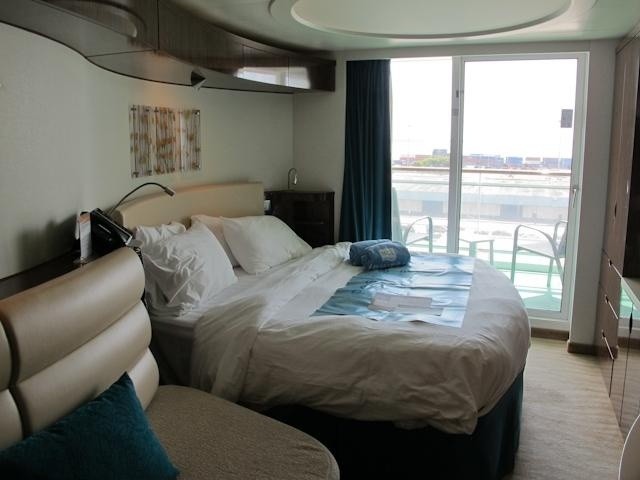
[[90, 208, 132, 254]]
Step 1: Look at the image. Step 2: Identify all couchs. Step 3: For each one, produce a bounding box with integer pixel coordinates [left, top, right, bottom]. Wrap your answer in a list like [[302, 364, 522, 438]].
[[0, 243, 343, 480]]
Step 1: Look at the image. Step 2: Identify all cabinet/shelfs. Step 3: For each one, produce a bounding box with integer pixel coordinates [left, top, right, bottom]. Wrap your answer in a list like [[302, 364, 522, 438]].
[[588, 19, 640, 397], [262, 185, 337, 248], [609, 283, 639, 442], [245, 38, 335, 92], [0, 1, 159, 59], [87, 2, 244, 96]]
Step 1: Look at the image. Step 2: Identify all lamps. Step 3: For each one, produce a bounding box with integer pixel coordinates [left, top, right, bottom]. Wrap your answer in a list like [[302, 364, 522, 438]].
[[189, 65, 207, 91], [108, 181, 176, 218], [287, 166, 298, 191]]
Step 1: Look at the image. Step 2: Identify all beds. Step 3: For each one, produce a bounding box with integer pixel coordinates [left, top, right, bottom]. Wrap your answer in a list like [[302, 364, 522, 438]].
[[110, 175, 534, 480]]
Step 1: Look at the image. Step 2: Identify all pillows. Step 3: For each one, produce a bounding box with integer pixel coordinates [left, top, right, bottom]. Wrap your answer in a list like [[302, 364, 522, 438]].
[[141, 219, 239, 315], [133, 219, 188, 305], [188, 213, 244, 269], [1, 368, 180, 479], [218, 212, 314, 276]]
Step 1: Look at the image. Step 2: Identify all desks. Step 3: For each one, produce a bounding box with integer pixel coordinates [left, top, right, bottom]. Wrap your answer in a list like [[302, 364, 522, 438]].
[[455, 231, 495, 265]]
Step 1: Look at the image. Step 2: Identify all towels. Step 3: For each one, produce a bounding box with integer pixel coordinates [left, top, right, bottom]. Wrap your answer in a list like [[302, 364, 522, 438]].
[[348, 237, 391, 267], [361, 241, 412, 269]]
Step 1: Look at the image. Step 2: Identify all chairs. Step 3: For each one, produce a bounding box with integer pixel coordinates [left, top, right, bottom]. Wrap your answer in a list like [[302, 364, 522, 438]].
[[388, 184, 436, 255], [509, 218, 568, 289], [616, 412, 639, 480]]
